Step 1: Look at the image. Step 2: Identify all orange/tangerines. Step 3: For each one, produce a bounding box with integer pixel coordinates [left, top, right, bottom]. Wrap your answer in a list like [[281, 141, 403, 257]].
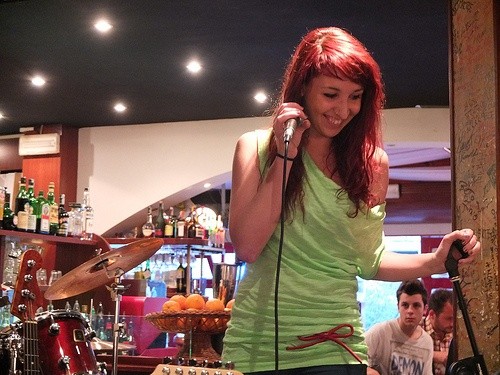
[[154, 293, 235, 331]]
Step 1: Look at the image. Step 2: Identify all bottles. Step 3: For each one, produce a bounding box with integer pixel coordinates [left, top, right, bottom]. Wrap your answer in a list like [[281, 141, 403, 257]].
[[64, 298, 133, 343], [0, 177, 95, 240], [141, 200, 225, 297]]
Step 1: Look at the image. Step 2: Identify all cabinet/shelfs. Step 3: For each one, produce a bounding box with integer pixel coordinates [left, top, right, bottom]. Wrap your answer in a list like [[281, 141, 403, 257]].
[[0, 229, 225, 375]]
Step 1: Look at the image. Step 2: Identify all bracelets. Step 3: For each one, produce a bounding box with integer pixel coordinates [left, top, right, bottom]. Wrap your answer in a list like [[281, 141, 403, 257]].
[[276, 153, 295, 161]]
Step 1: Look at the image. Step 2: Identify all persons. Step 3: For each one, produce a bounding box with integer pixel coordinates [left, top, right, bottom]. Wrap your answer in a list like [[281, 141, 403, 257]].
[[364, 278, 433, 375], [419, 289, 454, 375], [221, 27, 481, 375]]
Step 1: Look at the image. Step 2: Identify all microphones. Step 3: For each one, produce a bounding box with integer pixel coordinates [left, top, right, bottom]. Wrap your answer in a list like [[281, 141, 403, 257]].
[[284, 117, 301, 142]]
